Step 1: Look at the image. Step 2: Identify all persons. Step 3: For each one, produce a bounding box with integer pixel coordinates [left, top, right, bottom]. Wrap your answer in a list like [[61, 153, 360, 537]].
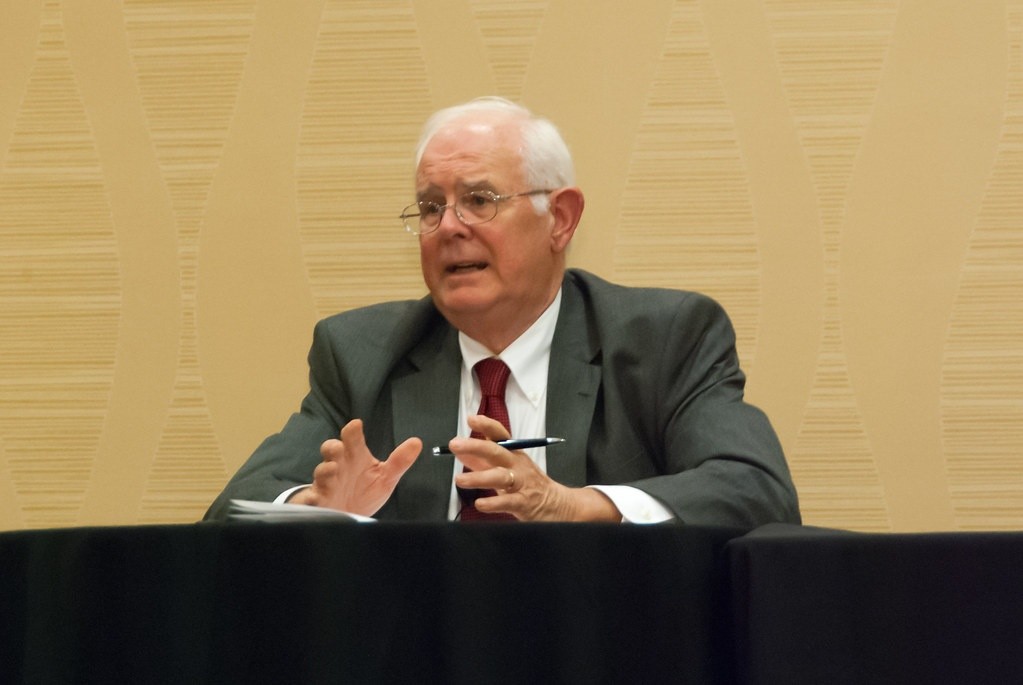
[[203, 96, 804, 525]]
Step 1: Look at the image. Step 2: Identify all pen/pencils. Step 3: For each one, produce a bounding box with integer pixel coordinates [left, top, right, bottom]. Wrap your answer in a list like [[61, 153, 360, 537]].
[[433, 438, 566, 456]]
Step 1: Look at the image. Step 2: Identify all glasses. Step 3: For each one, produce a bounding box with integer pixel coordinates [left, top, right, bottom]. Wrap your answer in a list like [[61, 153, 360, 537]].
[[400, 189, 553, 235]]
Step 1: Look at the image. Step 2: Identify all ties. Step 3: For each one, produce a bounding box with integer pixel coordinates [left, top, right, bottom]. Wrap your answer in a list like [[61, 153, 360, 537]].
[[461, 357, 519, 522]]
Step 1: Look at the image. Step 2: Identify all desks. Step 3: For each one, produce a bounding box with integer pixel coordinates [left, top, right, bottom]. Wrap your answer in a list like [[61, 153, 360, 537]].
[[0, 521, 1023, 685]]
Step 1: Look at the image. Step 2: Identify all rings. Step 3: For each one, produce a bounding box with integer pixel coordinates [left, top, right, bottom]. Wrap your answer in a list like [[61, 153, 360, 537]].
[[507, 473, 514, 491]]
